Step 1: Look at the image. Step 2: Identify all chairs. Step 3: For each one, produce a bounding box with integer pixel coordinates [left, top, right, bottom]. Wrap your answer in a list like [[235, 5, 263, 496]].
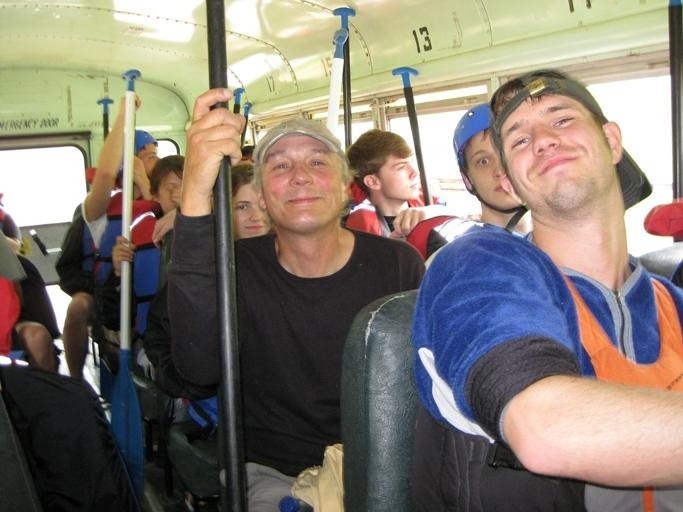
[[0, 393, 48, 511], [636, 238, 682, 280], [338, 288, 420, 512]]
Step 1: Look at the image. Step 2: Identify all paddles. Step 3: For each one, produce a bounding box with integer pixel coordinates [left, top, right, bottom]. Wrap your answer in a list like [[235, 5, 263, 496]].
[[110, 71, 143, 506]]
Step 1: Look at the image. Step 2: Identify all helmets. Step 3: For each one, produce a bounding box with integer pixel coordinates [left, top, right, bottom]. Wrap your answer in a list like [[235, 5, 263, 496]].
[[117, 130, 157, 175], [452, 102, 496, 172]]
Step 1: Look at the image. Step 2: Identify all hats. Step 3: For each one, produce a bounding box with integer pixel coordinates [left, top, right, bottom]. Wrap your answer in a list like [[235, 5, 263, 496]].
[[488, 67, 652, 212], [249, 117, 343, 167]]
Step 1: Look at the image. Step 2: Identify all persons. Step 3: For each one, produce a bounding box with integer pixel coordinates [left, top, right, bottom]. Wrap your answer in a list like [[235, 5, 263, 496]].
[[346, 129, 462, 240], [56, 94, 270, 435], [414, 68, 683, 512], [167, 87, 426, 512], [429, 101, 534, 256], [0, 209, 60, 373]]
